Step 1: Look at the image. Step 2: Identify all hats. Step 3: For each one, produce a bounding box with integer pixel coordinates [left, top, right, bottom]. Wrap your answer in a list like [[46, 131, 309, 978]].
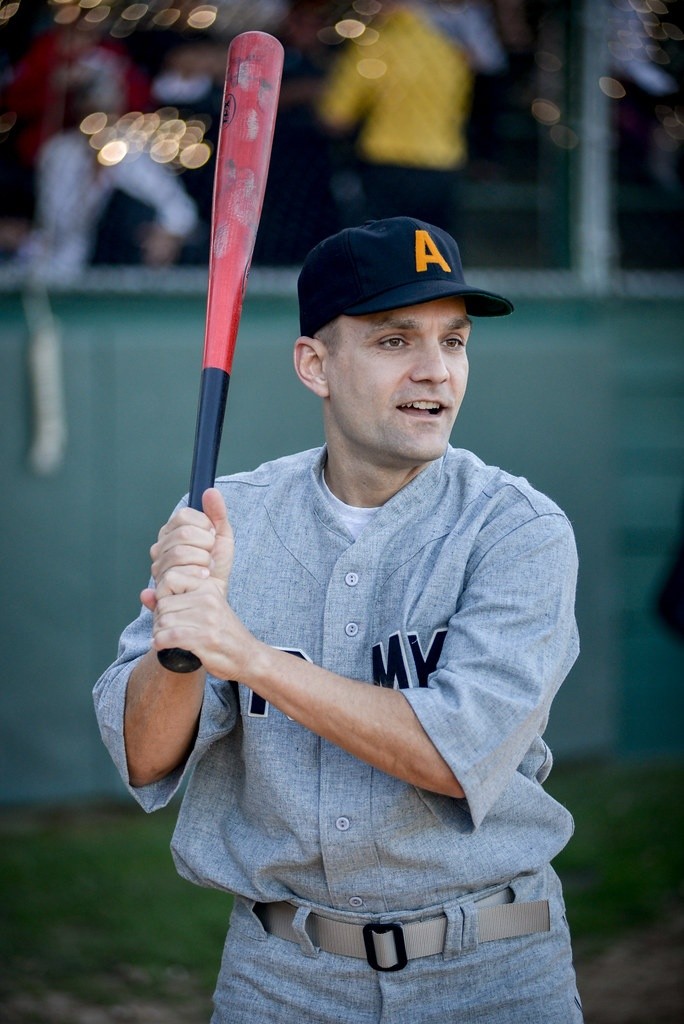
[[296, 216, 517, 335]]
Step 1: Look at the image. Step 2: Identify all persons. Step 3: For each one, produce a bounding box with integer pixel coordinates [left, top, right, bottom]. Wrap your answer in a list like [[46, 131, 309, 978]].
[[91, 216, 585, 1024], [0, 0, 683, 270]]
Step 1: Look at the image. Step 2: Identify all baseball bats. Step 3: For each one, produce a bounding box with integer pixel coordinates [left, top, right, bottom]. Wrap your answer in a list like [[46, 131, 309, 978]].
[[154, 30, 288, 675]]
